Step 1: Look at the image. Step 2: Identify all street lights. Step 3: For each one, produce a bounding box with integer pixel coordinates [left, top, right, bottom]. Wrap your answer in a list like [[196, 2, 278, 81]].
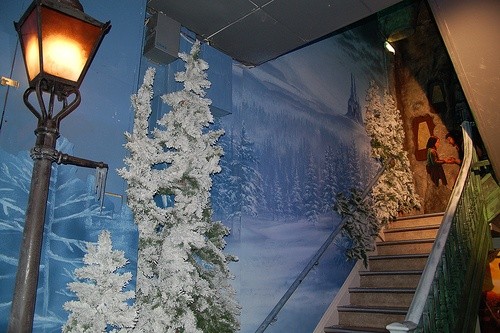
[[0, 1, 112, 333]]
